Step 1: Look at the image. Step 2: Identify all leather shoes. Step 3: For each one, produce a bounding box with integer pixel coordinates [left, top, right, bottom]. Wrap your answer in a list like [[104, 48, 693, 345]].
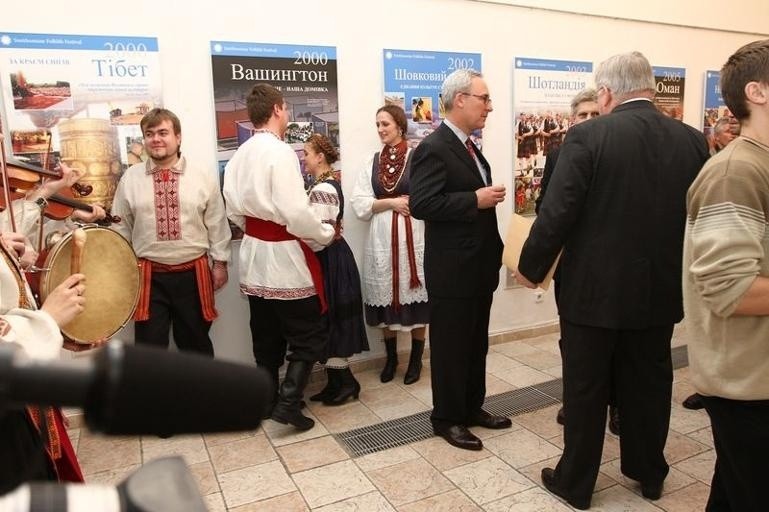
[[541, 467, 592, 510], [556, 401, 624, 436], [464, 407, 512, 429], [682, 392, 705, 411], [433, 418, 483, 450], [638, 476, 663, 500]]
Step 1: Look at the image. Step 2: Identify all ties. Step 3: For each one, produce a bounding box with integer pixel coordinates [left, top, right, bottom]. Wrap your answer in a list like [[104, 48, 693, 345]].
[[465, 139, 477, 161]]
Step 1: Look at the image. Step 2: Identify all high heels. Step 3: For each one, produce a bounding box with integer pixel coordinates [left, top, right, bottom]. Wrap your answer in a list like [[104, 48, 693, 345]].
[[309, 366, 361, 406]]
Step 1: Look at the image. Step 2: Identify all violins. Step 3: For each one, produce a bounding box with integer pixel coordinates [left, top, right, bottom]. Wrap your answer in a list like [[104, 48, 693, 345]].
[[0, 159, 92, 196], [0, 186, 121, 223]]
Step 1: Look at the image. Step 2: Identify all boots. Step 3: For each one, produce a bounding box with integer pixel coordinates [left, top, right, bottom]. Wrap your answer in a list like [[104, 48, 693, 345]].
[[272, 359, 315, 430], [404, 337, 425, 385], [255, 359, 306, 420], [380, 337, 399, 383]]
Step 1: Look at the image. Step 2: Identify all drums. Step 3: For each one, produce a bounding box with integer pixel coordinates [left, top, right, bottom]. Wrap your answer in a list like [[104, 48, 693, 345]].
[[29, 226, 142, 345]]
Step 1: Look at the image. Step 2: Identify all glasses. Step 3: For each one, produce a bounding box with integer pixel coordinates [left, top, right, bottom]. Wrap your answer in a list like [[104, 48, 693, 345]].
[[462, 92, 492, 105]]
